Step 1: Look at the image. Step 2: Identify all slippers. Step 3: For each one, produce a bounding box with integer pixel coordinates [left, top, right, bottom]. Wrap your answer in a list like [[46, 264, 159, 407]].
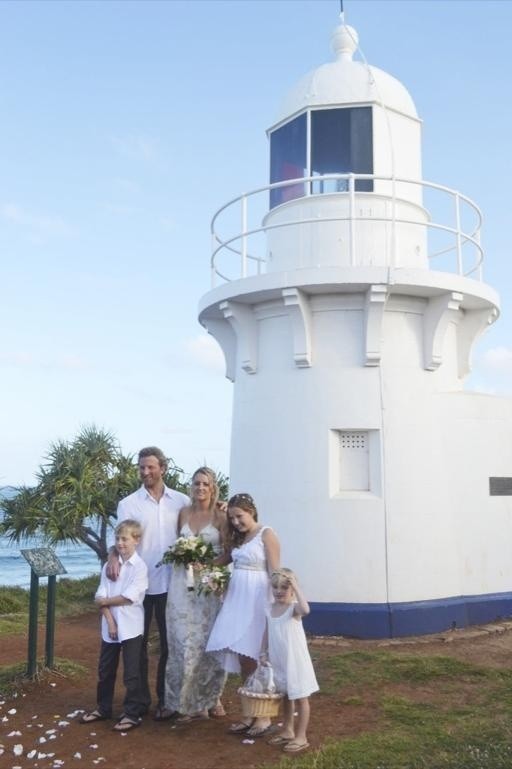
[[228, 719, 275, 737], [80, 708, 110, 724], [266, 735, 310, 752], [114, 713, 143, 731], [154, 702, 227, 725]]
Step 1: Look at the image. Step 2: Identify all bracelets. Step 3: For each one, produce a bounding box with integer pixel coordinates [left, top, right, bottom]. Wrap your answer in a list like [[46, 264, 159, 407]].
[[259, 653, 268, 657]]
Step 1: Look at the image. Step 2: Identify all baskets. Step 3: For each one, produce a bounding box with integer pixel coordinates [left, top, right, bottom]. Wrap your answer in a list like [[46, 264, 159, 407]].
[[237, 661, 286, 719]]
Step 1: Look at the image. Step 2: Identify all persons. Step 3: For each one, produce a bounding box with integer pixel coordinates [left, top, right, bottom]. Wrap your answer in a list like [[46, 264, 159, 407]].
[[260, 568, 320, 753], [166, 468, 235, 727], [107, 446, 193, 722], [79, 520, 148, 732], [205, 493, 281, 738]]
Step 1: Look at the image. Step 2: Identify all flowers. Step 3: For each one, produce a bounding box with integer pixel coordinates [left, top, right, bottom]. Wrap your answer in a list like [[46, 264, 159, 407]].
[[194, 567, 231, 600], [154, 534, 217, 568]]
